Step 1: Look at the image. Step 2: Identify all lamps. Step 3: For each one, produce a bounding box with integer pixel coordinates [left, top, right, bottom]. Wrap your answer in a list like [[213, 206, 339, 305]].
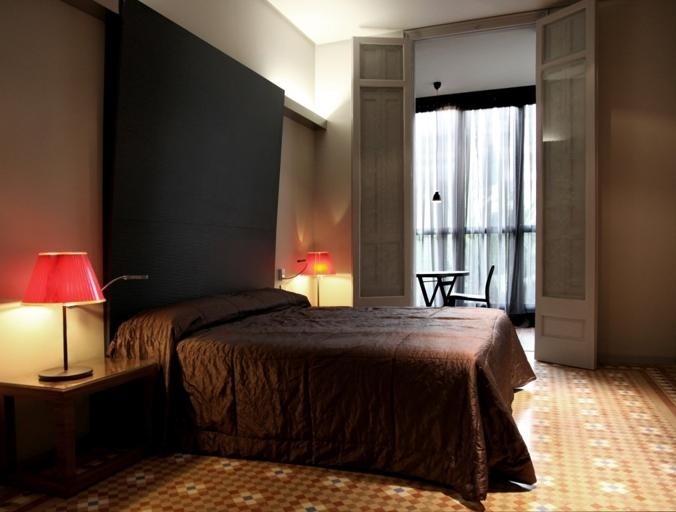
[[302, 250, 336, 308], [20, 249, 108, 381], [430, 81, 443, 203]]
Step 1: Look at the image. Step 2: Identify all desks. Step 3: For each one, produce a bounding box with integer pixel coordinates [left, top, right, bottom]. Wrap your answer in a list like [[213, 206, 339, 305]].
[[416, 271, 470, 307]]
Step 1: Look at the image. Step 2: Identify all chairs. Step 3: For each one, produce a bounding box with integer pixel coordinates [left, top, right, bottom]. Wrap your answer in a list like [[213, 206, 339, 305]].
[[449, 264, 495, 307]]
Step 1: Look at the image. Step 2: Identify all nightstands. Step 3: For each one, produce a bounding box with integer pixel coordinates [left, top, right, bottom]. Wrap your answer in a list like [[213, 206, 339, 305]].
[[0, 358, 160, 500]]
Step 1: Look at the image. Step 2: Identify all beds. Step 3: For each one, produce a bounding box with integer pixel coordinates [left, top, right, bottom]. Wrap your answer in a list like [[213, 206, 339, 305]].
[[115, 287, 518, 488]]
